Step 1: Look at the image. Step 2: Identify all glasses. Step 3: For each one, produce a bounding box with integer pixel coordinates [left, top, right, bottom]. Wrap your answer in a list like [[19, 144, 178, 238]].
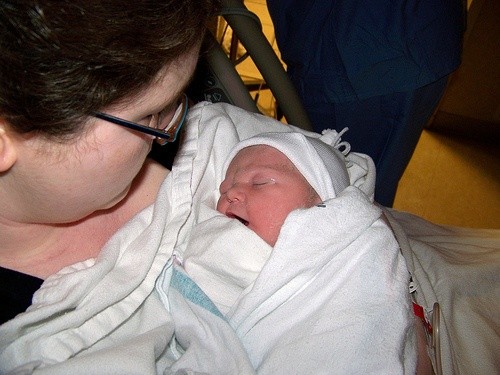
[[86, 89, 188, 145]]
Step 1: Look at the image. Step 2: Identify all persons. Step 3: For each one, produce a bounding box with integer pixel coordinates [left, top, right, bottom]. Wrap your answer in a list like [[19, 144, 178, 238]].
[[215, 131, 351, 249], [266, 0, 465, 209], [0, 0, 433, 375]]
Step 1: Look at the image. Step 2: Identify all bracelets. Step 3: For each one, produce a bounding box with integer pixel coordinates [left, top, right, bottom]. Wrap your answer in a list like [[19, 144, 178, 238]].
[[411, 302, 426, 327]]
